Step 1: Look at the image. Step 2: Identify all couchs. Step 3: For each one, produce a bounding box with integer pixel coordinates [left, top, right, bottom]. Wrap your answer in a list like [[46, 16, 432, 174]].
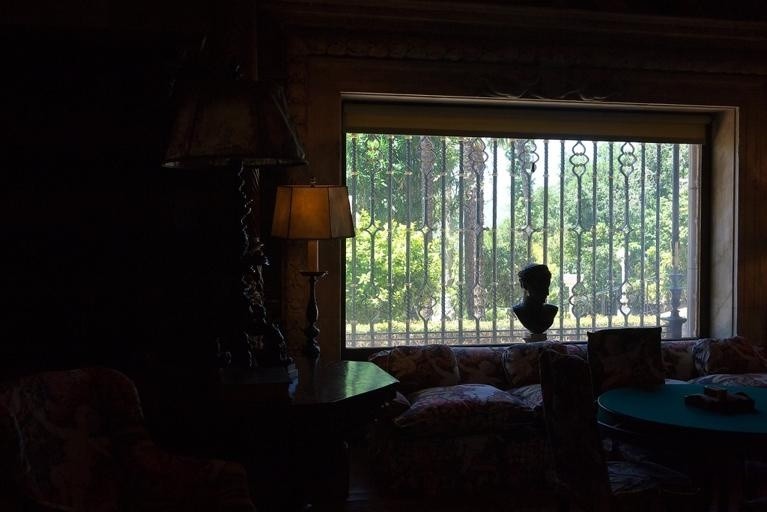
[[343, 339, 764, 510]]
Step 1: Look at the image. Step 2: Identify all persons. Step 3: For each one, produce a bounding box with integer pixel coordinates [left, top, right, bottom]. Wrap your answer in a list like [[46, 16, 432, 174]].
[[507, 263, 558, 344]]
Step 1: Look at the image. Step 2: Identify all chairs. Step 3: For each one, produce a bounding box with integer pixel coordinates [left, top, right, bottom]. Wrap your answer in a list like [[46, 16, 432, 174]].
[[0, 366, 256, 510]]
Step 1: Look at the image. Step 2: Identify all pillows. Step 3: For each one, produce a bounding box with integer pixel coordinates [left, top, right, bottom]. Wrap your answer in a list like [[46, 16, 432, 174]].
[[367, 327, 765, 497]]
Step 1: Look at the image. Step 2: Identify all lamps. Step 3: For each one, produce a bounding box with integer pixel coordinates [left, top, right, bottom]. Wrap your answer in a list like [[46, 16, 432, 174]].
[[269, 176, 355, 386]]
